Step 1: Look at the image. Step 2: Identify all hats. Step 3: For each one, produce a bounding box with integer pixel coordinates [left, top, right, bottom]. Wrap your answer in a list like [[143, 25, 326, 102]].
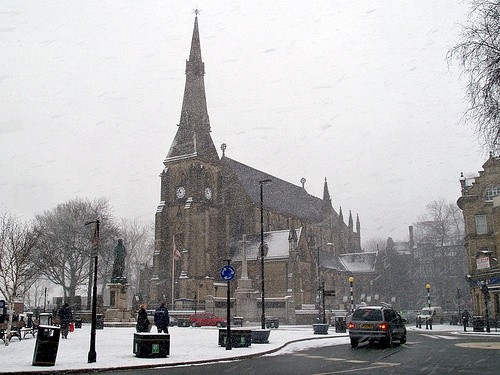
[[64, 303, 69, 307]]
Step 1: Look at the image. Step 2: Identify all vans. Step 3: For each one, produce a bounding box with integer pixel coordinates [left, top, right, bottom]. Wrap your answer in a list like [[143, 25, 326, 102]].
[[135, 310, 178, 326], [417, 306, 444, 324]]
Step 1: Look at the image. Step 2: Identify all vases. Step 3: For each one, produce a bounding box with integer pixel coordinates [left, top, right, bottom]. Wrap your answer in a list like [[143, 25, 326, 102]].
[[251, 329, 272, 342], [312, 324, 329, 334]]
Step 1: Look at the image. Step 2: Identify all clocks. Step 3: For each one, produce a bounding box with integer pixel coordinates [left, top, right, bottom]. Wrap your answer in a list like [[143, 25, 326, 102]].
[[176, 186, 186, 199], [204, 187, 212, 200]]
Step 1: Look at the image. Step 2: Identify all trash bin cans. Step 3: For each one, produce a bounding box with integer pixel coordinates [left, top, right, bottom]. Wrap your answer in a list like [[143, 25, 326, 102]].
[[96, 314, 103, 330], [335, 315, 347, 333], [32, 324, 61, 366], [472, 316, 484, 331], [233, 317, 243, 327]]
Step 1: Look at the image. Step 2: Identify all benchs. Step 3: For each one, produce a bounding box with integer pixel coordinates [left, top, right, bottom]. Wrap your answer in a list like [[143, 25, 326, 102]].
[[0, 321, 37, 343]]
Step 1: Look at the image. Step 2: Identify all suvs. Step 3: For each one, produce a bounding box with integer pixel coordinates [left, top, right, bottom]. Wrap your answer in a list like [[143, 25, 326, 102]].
[[349, 305, 407, 348]]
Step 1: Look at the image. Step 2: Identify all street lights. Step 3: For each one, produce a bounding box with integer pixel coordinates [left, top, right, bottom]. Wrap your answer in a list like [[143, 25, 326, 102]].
[[457, 288, 462, 324], [317, 242, 333, 324], [259, 179, 272, 329], [84, 218, 100, 363]]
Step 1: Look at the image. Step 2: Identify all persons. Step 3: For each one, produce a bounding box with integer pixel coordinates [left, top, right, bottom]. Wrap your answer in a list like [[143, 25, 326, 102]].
[[448, 310, 471, 327], [59, 302, 72, 339], [136, 304, 152, 332], [154, 303, 169, 333], [327, 310, 333, 326], [344, 308, 354, 316], [112, 238, 127, 277], [33, 317, 39, 329]]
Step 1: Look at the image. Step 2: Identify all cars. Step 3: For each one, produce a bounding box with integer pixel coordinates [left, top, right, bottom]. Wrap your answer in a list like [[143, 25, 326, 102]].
[[189, 312, 225, 327]]
